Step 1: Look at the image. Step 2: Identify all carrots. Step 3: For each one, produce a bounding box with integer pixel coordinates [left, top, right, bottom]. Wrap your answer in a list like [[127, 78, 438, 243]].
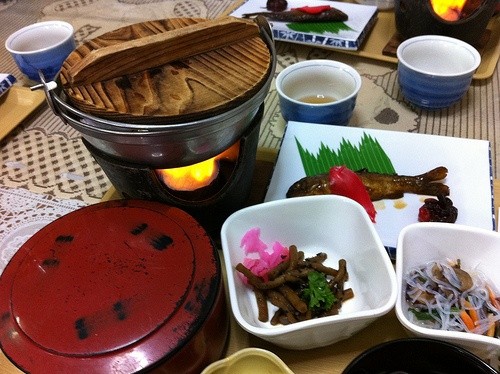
[[459, 287, 500, 339]]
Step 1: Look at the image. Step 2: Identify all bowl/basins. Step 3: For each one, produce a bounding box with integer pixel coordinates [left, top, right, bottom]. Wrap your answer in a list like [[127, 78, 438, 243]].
[[221, 194, 399, 350], [397, 221, 500, 346], [396, 34, 483, 111], [339, 336, 499, 374], [276, 59, 363, 128], [5, 20, 74, 83]]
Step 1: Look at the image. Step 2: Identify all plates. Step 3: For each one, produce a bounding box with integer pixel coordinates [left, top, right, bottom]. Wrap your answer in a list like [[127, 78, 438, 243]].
[[221, 0, 380, 52], [265, 121, 494, 247]]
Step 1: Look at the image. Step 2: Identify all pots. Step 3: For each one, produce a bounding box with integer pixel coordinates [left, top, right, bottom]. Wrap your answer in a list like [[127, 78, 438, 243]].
[[31, 15, 279, 167], [201, 347, 296, 373]]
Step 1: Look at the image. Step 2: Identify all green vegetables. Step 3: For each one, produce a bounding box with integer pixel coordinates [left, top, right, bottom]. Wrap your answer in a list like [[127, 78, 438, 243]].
[[406, 305, 460, 323], [300, 271, 338, 313]]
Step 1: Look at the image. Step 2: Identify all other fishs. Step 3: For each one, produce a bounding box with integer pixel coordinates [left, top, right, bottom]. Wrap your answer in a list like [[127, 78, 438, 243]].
[[284, 164, 451, 202], [241, 5, 348, 23]]
